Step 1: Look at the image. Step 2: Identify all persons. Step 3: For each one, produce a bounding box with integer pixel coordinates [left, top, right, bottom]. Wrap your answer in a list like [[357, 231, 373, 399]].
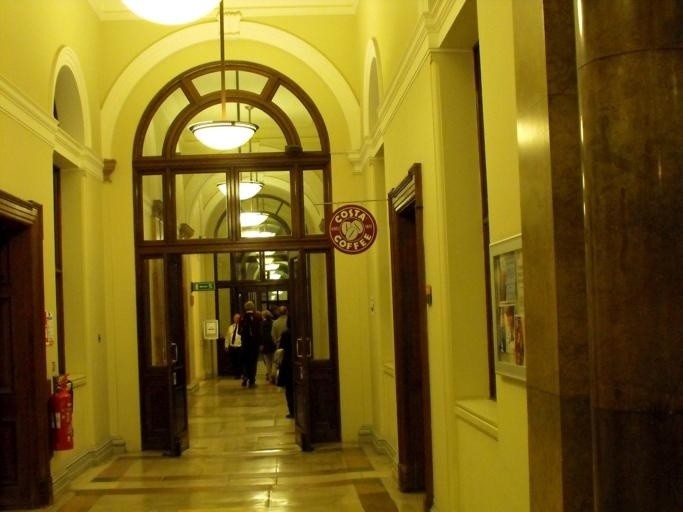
[[271, 319, 294, 418], [221, 297, 288, 387]]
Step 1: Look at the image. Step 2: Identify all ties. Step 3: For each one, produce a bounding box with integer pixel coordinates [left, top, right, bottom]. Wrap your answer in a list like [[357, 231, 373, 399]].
[[231, 324, 238, 345]]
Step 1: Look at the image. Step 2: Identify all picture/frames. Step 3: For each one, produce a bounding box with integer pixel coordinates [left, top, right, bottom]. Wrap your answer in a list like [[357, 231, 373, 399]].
[[487, 231, 526, 384]]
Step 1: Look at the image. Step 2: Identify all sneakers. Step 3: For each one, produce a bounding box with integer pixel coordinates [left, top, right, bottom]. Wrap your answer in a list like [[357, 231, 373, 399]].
[[249, 383, 257, 388], [240, 377, 248, 387]]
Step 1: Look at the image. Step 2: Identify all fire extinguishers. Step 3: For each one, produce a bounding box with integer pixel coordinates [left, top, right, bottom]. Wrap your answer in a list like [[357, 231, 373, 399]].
[[50, 373, 74, 450]]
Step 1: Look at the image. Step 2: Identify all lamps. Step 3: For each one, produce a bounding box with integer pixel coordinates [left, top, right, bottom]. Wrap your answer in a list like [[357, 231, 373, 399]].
[[188, 0, 262, 152], [215, 101, 283, 272]]
